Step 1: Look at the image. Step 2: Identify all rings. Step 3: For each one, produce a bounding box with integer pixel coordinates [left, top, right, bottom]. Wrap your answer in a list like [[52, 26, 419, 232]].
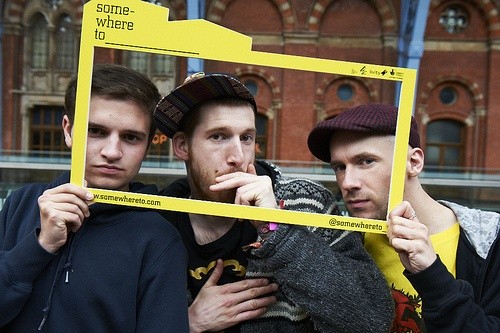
[[410, 208, 416, 219]]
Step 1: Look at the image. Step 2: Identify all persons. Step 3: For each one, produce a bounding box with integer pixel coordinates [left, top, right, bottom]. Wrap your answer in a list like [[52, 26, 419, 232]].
[[0, 63, 191, 332], [153, 70, 396, 333], [306, 101, 500, 333]]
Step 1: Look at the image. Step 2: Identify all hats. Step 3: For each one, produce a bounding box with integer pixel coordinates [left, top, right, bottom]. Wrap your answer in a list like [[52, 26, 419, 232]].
[[306, 103, 422, 164], [151, 71, 258, 139]]
